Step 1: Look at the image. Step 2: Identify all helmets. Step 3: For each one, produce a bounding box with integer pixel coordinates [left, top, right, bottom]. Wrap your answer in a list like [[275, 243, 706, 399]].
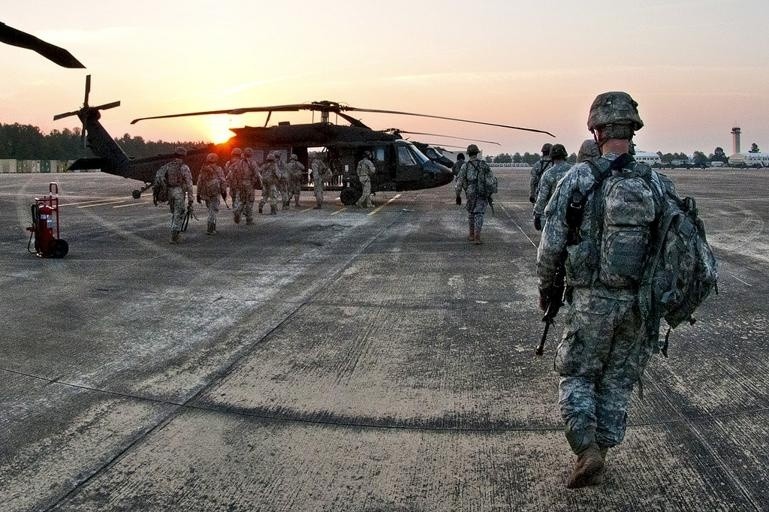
[[466, 145, 480, 154], [587, 92, 643, 130], [207, 153, 219, 163], [175, 147, 187, 157], [231, 148, 297, 160], [542, 143, 567, 158]]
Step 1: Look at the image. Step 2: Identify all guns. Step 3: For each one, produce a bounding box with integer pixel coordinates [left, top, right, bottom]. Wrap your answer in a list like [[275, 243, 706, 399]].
[[489, 197, 495, 215], [536, 189, 586, 357], [221, 192, 230, 208]]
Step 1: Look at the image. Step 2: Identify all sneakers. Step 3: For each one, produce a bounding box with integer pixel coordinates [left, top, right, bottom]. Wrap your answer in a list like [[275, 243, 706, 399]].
[[567, 451, 604, 485]]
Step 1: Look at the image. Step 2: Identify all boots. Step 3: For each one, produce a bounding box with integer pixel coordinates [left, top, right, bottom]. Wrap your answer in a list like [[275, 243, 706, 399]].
[[468, 227, 482, 244], [233, 214, 256, 224], [170, 229, 185, 243], [207, 222, 219, 235]]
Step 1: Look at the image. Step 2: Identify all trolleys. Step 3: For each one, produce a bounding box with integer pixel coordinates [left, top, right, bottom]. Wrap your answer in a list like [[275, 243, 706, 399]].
[[25, 182, 69, 258]]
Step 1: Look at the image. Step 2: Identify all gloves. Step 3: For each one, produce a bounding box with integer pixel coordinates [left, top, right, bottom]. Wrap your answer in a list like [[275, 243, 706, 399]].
[[534, 217, 541, 230]]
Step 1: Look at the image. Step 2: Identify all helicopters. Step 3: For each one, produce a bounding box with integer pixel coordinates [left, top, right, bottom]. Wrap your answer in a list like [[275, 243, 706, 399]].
[[375, 127, 502, 169], [53, 74, 558, 199]]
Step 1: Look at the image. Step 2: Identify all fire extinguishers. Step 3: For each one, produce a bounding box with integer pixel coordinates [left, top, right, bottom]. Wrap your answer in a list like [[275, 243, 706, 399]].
[[37, 196, 53, 244]]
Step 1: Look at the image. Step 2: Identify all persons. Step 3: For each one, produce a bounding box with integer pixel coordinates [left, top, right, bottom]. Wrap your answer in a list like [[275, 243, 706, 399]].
[[311, 151, 326, 210], [454, 144, 492, 245], [532, 144, 576, 229], [529, 143, 552, 204], [354, 150, 376, 210], [154, 145, 306, 243], [536, 92, 681, 488], [452, 153, 467, 205]]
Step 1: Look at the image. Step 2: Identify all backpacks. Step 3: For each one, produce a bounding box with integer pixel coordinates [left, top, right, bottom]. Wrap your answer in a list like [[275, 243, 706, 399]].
[[478, 168, 497, 196], [590, 158, 654, 287], [153, 170, 168, 205], [639, 192, 719, 327]]
